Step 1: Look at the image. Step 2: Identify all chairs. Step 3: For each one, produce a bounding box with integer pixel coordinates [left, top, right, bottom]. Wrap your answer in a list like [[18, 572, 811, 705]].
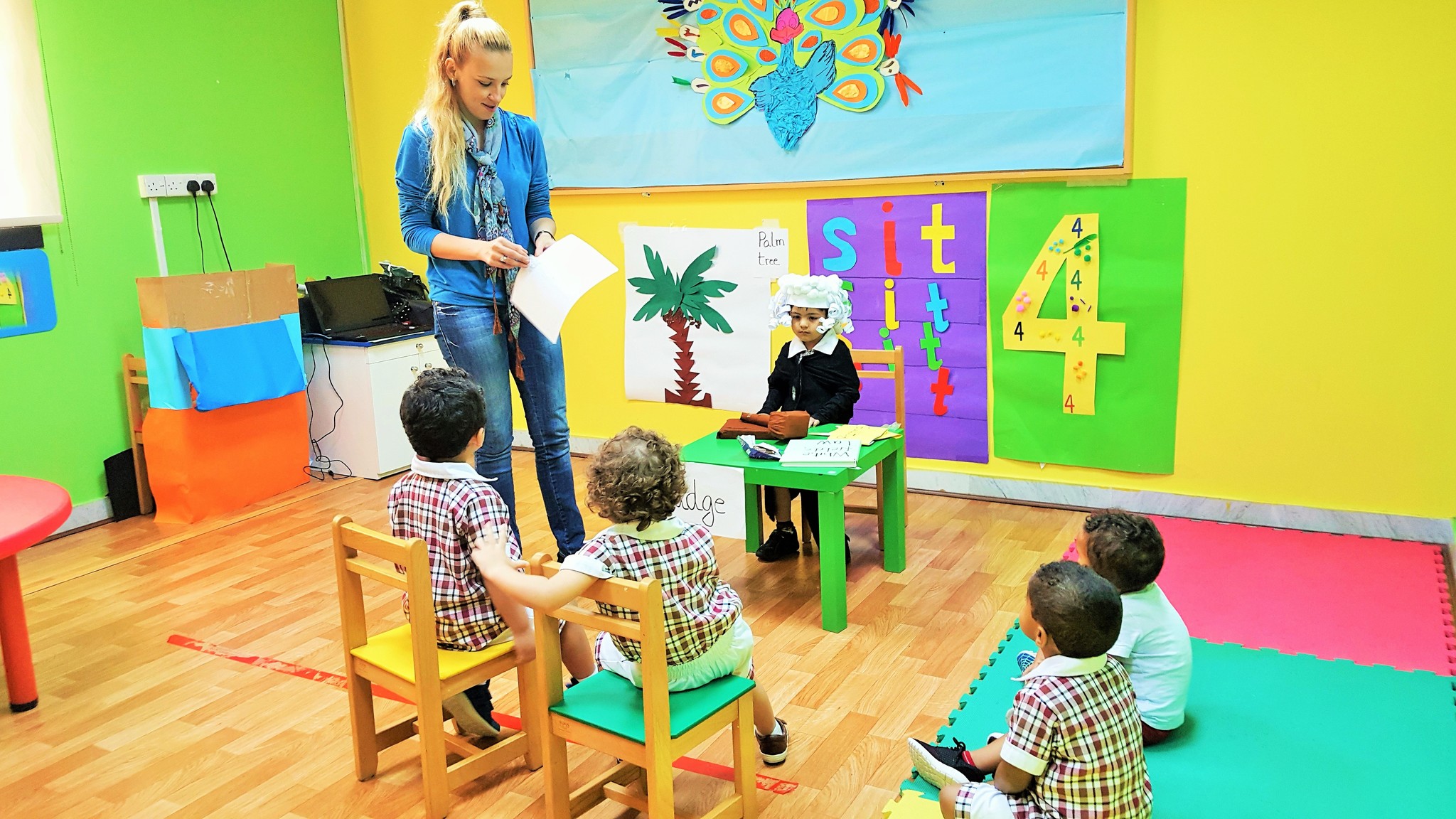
[[124, 354, 198, 514], [531, 552, 758, 819], [332, 513, 542, 818], [801, 343, 905, 554]]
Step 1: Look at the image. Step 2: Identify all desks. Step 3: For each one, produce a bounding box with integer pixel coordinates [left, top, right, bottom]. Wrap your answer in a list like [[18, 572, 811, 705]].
[[0, 474, 73, 717], [675, 418, 907, 633]]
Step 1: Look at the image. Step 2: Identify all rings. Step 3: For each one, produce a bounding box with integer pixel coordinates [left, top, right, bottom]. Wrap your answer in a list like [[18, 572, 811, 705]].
[[500, 255, 508, 263]]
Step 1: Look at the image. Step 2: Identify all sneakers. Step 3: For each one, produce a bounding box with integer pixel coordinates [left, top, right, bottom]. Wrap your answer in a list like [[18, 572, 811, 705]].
[[442, 679, 500, 737], [987, 733, 1004, 746], [1016, 650, 1037, 674], [845, 533, 851, 565], [564, 677, 579, 690], [907, 737, 986, 790], [755, 717, 790, 764], [755, 528, 799, 559]]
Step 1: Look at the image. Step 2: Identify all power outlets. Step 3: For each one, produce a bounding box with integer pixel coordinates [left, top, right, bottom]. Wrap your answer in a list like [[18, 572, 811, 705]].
[[138, 173, 218, 199]]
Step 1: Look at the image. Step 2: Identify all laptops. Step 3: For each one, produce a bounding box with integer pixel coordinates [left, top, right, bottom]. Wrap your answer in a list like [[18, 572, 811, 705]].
[[305, 274, 432, 343]]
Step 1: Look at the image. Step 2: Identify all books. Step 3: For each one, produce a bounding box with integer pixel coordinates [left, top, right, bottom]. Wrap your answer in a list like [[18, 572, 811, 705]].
[[778, 437, 862, 470]]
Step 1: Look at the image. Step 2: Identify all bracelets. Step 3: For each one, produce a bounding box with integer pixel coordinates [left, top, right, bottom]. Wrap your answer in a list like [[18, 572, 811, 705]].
[[534, 230, 556, 245]]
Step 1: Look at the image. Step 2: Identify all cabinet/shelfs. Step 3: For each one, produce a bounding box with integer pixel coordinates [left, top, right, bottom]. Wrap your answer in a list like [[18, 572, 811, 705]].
[[301, 327, 456, 479]]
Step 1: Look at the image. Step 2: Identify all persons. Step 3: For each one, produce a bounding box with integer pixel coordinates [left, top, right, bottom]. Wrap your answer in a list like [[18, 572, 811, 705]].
[[754, 272, 862, 573], [906, 557, 1157, 819], [384, 364, 601, 740], [470, 422, 792, 768], [1014, 506, 1195, 752], [392, 0, 596, 569]]
